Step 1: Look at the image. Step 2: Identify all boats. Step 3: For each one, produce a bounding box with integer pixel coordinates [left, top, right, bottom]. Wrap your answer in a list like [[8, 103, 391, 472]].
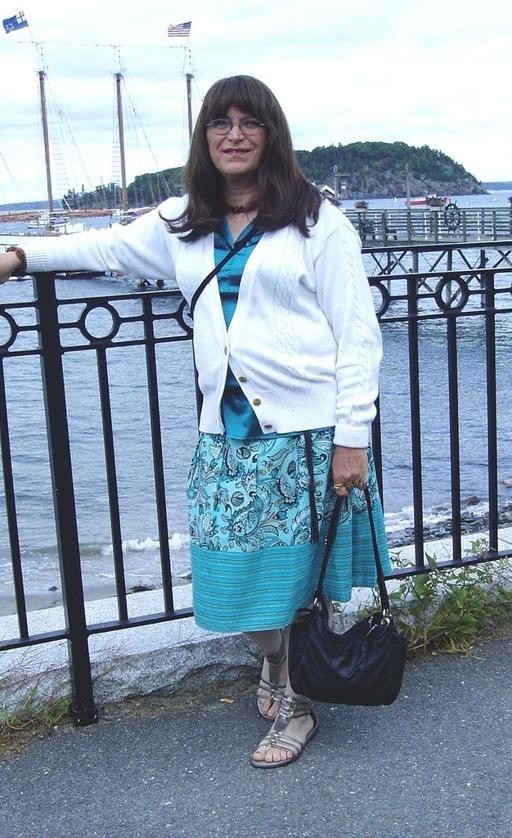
[[404, 192, 448, 209]]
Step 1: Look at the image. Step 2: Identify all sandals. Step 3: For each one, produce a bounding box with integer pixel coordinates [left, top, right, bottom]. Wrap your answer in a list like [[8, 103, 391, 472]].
[[251, 694, 318, 769], [254, 629, 293, 721]]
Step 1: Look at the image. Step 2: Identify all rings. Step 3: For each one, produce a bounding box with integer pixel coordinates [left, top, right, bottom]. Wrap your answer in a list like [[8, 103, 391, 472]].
[[346, 484, 353, 492], [333, 483, 344, 491]]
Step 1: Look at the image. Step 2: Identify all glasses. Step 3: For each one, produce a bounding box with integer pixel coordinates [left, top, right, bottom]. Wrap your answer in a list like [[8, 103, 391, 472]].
[[204, 118, 265, 134]]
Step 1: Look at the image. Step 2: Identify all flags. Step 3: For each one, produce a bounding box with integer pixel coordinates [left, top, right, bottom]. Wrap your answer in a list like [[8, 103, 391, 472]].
[[167, 21, 191, 37], [3, 10, 28, 34]]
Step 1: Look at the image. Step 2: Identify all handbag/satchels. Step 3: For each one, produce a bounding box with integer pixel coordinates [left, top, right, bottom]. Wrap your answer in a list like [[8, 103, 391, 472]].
[[288, 484, 405, 706]]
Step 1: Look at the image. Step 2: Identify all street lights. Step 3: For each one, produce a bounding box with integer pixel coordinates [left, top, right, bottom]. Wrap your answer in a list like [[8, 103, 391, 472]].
[[406, 163, 411, 209], [332, 164, 339, 200]]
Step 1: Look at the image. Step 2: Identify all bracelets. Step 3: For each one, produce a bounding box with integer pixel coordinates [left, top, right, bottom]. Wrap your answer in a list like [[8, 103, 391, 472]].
[[5, 246, 27, 278]]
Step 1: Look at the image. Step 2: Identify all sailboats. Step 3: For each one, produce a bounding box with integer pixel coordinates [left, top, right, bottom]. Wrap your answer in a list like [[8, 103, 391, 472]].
[[95, 43, 177, 229], [1, 9, 105, 235]]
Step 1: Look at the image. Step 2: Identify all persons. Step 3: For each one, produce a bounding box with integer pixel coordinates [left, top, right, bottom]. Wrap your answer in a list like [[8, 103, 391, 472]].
[[0, 75, 385, 769]]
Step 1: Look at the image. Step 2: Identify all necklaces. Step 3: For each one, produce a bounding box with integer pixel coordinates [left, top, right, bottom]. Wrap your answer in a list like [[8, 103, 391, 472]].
[[223, 198, 261, 213]]
